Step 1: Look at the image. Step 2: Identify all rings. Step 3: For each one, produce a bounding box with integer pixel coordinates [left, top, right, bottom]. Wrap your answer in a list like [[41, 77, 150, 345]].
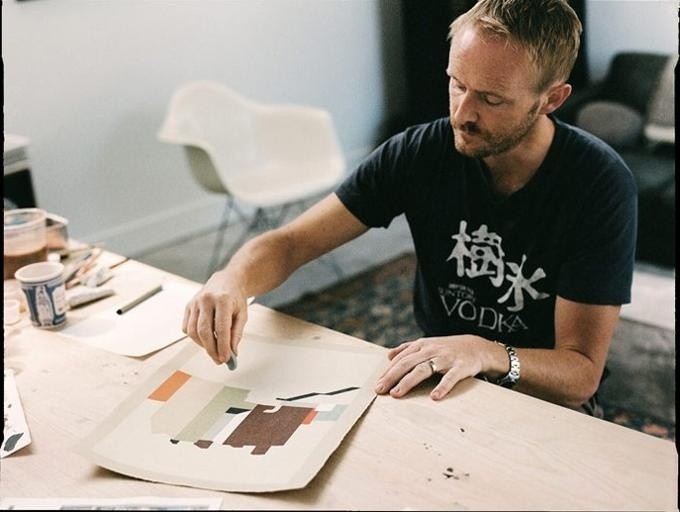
[[426, 360, 437, 374]]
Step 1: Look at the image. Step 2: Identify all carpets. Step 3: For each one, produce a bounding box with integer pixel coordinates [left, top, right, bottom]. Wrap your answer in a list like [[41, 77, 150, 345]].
[[273, 249, 678, 443]]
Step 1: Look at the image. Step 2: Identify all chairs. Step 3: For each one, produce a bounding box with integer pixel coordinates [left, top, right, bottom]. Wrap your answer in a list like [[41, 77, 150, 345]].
[[158, 80, 348, 293], [556, 51, 680, 271]]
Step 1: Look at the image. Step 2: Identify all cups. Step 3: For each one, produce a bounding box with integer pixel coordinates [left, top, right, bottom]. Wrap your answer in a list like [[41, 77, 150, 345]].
[[14, 260, 69, 331], [4, 206, 50, 282]]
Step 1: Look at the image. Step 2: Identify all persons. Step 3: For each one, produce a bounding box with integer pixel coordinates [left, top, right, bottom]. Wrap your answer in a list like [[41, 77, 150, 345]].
[[182, 2, 638, 422]]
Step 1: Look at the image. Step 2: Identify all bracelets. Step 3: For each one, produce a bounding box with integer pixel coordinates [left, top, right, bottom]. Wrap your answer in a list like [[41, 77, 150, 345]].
[[484, 340, 520, 390]]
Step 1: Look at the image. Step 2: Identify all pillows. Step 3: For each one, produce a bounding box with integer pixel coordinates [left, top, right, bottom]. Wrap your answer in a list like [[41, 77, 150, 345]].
[[575, 100, 643, 150]]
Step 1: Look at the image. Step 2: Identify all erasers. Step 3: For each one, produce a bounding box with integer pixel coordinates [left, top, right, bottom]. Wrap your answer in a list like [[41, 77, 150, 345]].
[[214, 317, 237, 370]]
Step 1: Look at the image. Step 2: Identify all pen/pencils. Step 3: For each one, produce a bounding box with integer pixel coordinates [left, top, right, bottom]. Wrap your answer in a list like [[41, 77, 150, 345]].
[[58, 243, 131, 289], [115, 285, 164, 315]]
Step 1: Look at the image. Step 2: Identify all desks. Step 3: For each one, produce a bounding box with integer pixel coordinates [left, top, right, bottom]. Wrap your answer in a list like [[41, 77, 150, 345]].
[[0, 237, 680, 512]]
